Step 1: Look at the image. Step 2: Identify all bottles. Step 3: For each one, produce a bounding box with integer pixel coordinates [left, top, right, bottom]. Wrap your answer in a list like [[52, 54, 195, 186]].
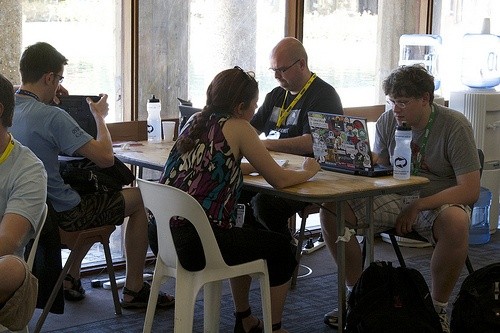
[[464, 182, 493, 245], [459, 32, 500, 90], [393, 121, 413, 180], [147, 94, 162, 143], [396, 35, 442, 91]]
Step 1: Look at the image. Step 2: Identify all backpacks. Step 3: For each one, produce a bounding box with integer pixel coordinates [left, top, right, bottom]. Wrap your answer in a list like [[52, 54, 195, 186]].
[[453, 261, 500, 333], [343, 261, 443, 333]]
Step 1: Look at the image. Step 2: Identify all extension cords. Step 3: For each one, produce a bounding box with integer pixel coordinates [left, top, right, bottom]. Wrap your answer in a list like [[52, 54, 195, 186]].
[[303, 241, 326, 255]]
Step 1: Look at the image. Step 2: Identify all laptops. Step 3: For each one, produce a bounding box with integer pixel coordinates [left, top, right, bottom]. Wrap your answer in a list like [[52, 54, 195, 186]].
[[308, 112, 396, 177], [179, 105, 203, 121]]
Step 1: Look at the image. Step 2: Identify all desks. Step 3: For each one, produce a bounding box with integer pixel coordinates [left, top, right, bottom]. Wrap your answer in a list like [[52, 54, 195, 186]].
[[91, 139, 430, 333]]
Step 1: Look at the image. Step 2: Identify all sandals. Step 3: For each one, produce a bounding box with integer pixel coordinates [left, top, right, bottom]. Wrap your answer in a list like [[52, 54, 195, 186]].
[[120, 282, 175, 309], [63, 274, 85, 298], [233, 307, 281, 333]]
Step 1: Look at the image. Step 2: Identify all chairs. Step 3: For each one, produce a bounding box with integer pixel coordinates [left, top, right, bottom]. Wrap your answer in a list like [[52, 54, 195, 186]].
[[0, 203, 48, 333], [32, 197, 122, 333], [362, 149, 485, 274], [137, 177, 273, 333]]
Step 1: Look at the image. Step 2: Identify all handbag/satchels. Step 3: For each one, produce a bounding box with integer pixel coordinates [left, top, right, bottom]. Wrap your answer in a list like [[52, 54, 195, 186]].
[[60, 152, 135, 188]]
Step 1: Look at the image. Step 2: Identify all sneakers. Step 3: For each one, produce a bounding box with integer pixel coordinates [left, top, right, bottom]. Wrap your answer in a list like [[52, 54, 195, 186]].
[[433, 300, 455, 333], [324, 291, 352, 328]]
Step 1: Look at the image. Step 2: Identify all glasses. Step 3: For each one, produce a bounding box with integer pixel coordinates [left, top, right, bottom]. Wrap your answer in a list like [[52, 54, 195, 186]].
[[269, 58, 300, 73], [234, 66, 258, 87], [386, 97, 415, 108], [45, 72, 64, 83]]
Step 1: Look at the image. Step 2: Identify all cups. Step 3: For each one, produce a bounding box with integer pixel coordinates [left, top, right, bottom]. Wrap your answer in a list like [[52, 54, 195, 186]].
[[161, 121, 177, 142]]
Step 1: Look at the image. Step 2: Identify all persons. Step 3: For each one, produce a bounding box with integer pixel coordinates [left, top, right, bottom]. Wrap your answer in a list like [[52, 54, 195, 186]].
[[147, 66, 321, 333], [6, 42, 174, 309], [255, 37, 344, 257], [319, 66, 481, 333], [0, 75, 48, 333]]
